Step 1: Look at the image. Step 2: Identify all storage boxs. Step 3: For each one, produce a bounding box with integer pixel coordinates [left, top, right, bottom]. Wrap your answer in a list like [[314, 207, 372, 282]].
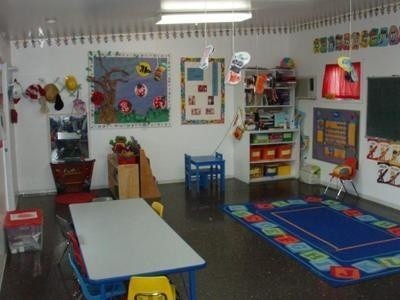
[[4, 208, 44, 255]]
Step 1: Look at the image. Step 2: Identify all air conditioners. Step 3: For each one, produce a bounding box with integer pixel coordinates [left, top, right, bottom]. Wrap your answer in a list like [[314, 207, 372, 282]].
[[295, 75, 316, 99]]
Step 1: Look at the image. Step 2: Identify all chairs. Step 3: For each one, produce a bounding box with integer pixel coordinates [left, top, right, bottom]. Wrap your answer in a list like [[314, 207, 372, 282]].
[[56, 196, 176, 300], [208, 152, 222, 183], [324, 157, 359, 199], [184, 154, 200, 190]]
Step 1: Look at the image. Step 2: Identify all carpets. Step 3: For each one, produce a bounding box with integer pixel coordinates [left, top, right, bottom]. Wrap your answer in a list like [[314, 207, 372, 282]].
[[56, 192, 96, 205], [218, 195, 400, 288]]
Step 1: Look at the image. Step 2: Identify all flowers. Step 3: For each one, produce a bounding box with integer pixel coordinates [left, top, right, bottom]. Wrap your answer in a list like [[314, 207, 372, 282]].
[[109, 135, 141, 160]]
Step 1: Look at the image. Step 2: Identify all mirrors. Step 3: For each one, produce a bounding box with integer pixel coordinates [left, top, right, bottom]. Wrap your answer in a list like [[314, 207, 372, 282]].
[[48, 114, 90, 160]]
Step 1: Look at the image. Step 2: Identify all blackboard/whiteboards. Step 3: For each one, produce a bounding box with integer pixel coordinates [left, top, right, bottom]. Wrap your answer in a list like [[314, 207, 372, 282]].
[[365, 75, 400, 144]]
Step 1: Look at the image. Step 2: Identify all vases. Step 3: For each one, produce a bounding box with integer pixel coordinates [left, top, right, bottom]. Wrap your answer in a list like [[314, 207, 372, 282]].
[[117, 155, 136, 164]]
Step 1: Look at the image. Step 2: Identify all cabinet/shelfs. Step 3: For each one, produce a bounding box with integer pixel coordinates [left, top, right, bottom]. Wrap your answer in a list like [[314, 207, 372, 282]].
[[107, 154, 139, 200], [139, 149, 162, 198], [49, 158, 96, 194], [233, 68, 301, 184]]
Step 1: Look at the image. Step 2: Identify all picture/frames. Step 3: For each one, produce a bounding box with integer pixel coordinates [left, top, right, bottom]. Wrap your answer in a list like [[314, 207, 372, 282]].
[[180, 57, 225, 125], [88, 51, 172, 129]]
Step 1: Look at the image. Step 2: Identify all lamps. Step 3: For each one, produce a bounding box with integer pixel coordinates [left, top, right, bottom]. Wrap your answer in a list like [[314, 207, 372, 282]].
[[153, 8, 257, 26]]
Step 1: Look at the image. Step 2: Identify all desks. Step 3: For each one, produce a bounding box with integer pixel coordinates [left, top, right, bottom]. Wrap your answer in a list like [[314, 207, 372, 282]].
[[190, 156, 225, 191], [69, 198, 206, 300], [57, 131, 80, 157]]
